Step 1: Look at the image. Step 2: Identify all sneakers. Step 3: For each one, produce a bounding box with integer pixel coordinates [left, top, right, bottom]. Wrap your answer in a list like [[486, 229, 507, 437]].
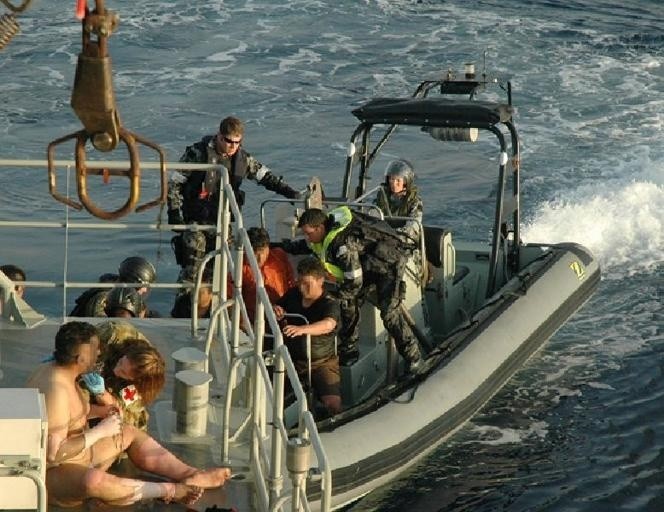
[[398, 356, 426, 382]]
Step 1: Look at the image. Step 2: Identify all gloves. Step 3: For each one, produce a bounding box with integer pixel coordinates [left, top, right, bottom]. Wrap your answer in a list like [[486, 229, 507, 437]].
[[81, 373, 105, 394]]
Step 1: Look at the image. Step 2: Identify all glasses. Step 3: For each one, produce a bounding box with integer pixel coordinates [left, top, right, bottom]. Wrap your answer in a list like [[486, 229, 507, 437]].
[[224, 137, 242, 143]]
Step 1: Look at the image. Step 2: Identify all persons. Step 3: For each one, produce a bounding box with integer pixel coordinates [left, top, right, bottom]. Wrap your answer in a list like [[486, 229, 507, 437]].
[[65, 256, 163, 318], [367, 157, 423, 285], [265, 256, 343, 417], [0, 264, 26, 316], [169, 268, 213, 319], [224, 226, 299, 334], [103, 283, 147, 319], [166, 114, 302, 290], [22, 320, 232, 510], [283, 202, 427, 385], [74, 318, 167, 461]]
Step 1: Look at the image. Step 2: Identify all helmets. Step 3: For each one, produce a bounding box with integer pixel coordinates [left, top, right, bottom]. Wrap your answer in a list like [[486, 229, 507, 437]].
[[384, 159, 414, 185], [119, 257, 156, 283]]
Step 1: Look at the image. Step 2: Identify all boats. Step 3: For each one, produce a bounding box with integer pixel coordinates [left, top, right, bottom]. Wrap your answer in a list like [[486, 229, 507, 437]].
[[0, 143, 340, 512], [262, 55, 606, 512]]
